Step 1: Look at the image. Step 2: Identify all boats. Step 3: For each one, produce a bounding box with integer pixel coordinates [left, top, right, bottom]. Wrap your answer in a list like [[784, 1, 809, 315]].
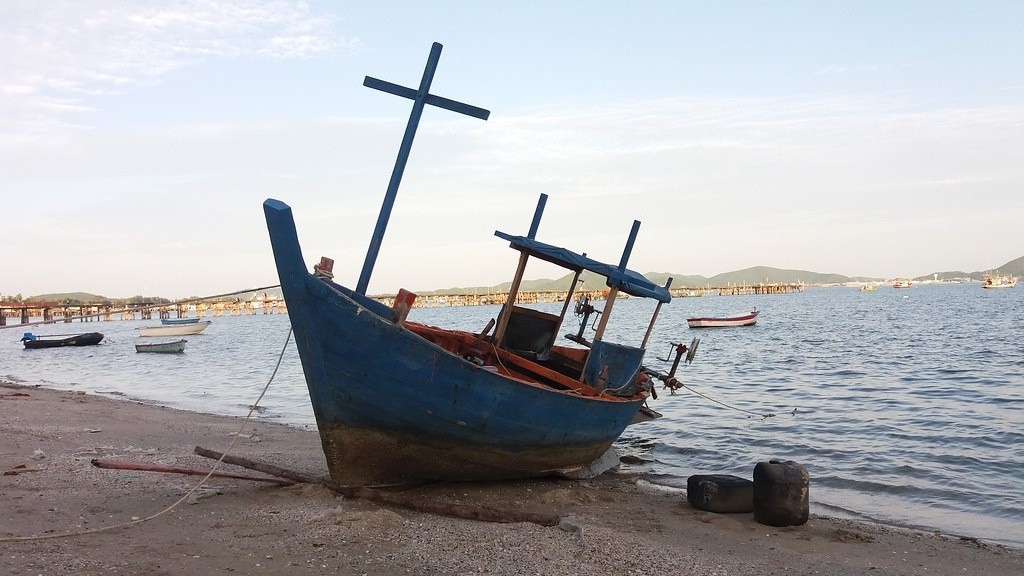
[[932, 278, 963, 284], [263, 41, 699, 491], [20, 332, 104, 350], [136, 339, 187, 352], [615, 290, 629, 299], [893, 278, 913, 288], [860, 285, 879, 291], [138, 320, 213, 336], [687, 306, 762, 328], [161, 317, 200, 325], [982, 269, 1018, 288], [670, 286, 704, 298]]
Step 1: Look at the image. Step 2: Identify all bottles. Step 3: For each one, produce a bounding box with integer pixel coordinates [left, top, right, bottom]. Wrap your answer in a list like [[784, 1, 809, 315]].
[[479, 366, 498, 373]]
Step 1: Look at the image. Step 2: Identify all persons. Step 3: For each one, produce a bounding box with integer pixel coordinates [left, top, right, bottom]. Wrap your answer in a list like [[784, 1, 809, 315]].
[[67, 298, 70, 304], [263, 292, 267, 299], [987, 276, 992, 285]]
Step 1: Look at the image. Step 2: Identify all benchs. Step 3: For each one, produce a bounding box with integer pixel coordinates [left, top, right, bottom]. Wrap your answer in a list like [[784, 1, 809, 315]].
[[550, 350, 645, 392], [466, 333, 619, 401]]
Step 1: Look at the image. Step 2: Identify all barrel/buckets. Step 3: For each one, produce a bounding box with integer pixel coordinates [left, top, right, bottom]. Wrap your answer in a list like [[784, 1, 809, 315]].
[[685, 473, 754, 515], [751, 459, 810, 527]]
